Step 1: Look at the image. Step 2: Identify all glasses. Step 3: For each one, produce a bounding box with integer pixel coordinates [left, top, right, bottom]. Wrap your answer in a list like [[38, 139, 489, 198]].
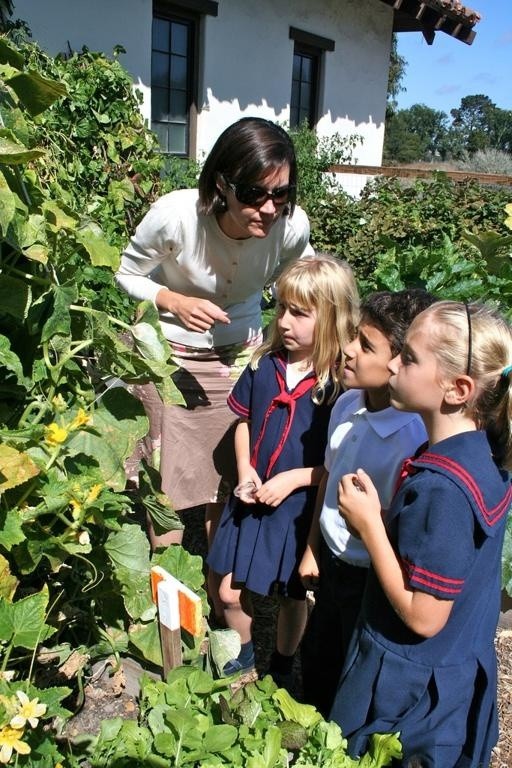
[[218, 171, 298, 209]]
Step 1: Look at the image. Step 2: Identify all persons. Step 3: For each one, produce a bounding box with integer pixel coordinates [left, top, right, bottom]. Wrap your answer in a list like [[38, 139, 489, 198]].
[[297, 287, 448, 724], [203, 253, 361, 692], [324, 300, 512, 768], [111, 118, 317, 634]]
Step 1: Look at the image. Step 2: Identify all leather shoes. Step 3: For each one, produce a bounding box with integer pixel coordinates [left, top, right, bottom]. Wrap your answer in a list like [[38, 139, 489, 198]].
[[217, 651, 259, 680]]
